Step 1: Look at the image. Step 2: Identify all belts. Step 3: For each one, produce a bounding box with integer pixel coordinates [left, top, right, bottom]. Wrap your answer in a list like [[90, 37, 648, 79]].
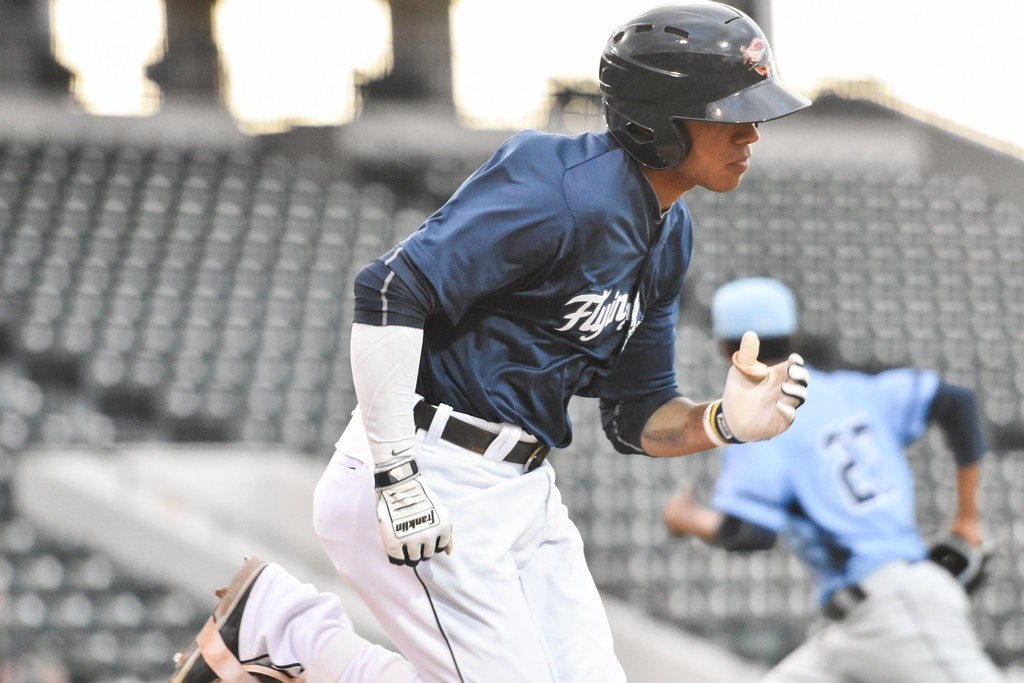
[[823, 584, 866, 621], [414, 400, 552, 473]]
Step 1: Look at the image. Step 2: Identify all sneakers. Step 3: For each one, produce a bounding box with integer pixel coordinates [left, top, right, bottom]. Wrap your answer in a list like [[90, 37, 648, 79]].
[[167, 555, 305, 683]]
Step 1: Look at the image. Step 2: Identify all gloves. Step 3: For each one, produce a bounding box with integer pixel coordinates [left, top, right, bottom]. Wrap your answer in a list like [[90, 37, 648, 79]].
[[372, 460, 451, 566], [703, 330, 809, 448]]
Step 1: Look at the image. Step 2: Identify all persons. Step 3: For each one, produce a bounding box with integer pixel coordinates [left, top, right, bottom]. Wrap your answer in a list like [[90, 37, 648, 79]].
[[663, 278, 1005, 683], [167, 1, 810, 683]]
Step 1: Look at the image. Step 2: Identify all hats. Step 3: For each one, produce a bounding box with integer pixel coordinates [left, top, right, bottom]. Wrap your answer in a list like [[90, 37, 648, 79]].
[[711, 276, 796, 343]]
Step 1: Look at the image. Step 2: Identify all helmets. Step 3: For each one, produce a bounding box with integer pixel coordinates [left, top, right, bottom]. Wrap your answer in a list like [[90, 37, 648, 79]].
[[600, 2, 813, 171]]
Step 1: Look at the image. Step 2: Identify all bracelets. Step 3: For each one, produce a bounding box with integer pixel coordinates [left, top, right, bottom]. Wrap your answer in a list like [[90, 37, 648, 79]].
[[703, 398, 732, 447]]
